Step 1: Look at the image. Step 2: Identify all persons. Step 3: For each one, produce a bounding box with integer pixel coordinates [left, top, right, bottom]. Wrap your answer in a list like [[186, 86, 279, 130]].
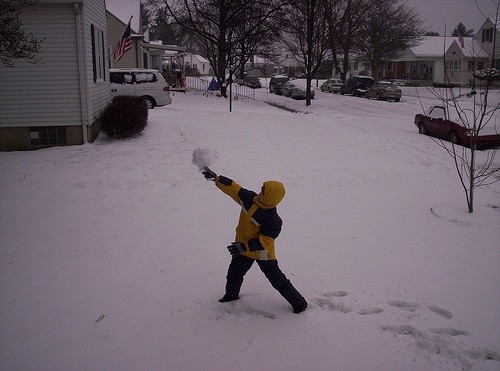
[[201, 164, 307, 314]]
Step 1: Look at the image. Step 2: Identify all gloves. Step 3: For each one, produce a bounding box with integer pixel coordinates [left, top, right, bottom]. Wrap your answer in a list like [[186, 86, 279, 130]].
[[202, 166, 217, 182], [227, 242, 247, 257]]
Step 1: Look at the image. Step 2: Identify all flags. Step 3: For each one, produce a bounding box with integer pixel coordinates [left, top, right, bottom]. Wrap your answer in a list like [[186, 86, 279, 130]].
[[113, 20, 133, 61]]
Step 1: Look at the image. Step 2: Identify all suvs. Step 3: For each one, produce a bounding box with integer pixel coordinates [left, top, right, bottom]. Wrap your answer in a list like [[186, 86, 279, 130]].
[[109, 68, 172, 109], [340, 75, 375, 97], [236, 72, 262, 89]]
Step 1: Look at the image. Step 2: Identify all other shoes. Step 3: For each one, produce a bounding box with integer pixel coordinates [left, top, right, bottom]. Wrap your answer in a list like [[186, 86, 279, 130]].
[[218, 295, 240, 303], [293, 302, 307, 314]]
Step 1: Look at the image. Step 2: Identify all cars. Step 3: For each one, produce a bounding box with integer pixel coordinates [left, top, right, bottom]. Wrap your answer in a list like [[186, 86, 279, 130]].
[[364, 81, 402, 102], [320, 79, 346, 94], [268, 73, 316, 101], [413, 104, 500, 150]]
